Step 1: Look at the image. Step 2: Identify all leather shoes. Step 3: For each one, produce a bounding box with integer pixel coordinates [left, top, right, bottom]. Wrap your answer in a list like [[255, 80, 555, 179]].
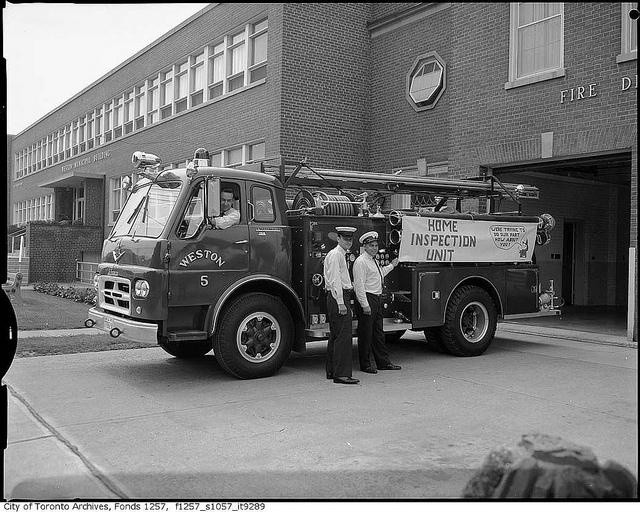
[[377, 364, 401, 370], [326, 372, 360, 384], [360, 367, 377, 374]]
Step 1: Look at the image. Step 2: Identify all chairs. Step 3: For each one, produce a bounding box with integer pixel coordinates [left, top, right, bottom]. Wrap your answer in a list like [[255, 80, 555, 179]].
[[1, 271, 24, 302]]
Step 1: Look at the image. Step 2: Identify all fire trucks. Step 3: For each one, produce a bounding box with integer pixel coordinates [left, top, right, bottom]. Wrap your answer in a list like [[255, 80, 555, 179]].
[[78, 147, 565, 381]]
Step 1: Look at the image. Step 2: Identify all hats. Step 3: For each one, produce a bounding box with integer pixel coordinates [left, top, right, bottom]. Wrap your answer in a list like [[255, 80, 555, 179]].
[[358, 230, 379, 244], [334, 226, 358, 237]]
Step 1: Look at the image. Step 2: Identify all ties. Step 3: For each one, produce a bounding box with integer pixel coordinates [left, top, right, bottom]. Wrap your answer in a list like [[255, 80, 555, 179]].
[[220, 212, 225, 217], [373, 257, 382, 279]]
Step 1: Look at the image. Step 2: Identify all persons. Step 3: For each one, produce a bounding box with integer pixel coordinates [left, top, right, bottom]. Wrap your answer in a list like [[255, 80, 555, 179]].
[[321, 224, 361, 386], [351, 230, 402, 375], [208, 184, 243, 233]]
